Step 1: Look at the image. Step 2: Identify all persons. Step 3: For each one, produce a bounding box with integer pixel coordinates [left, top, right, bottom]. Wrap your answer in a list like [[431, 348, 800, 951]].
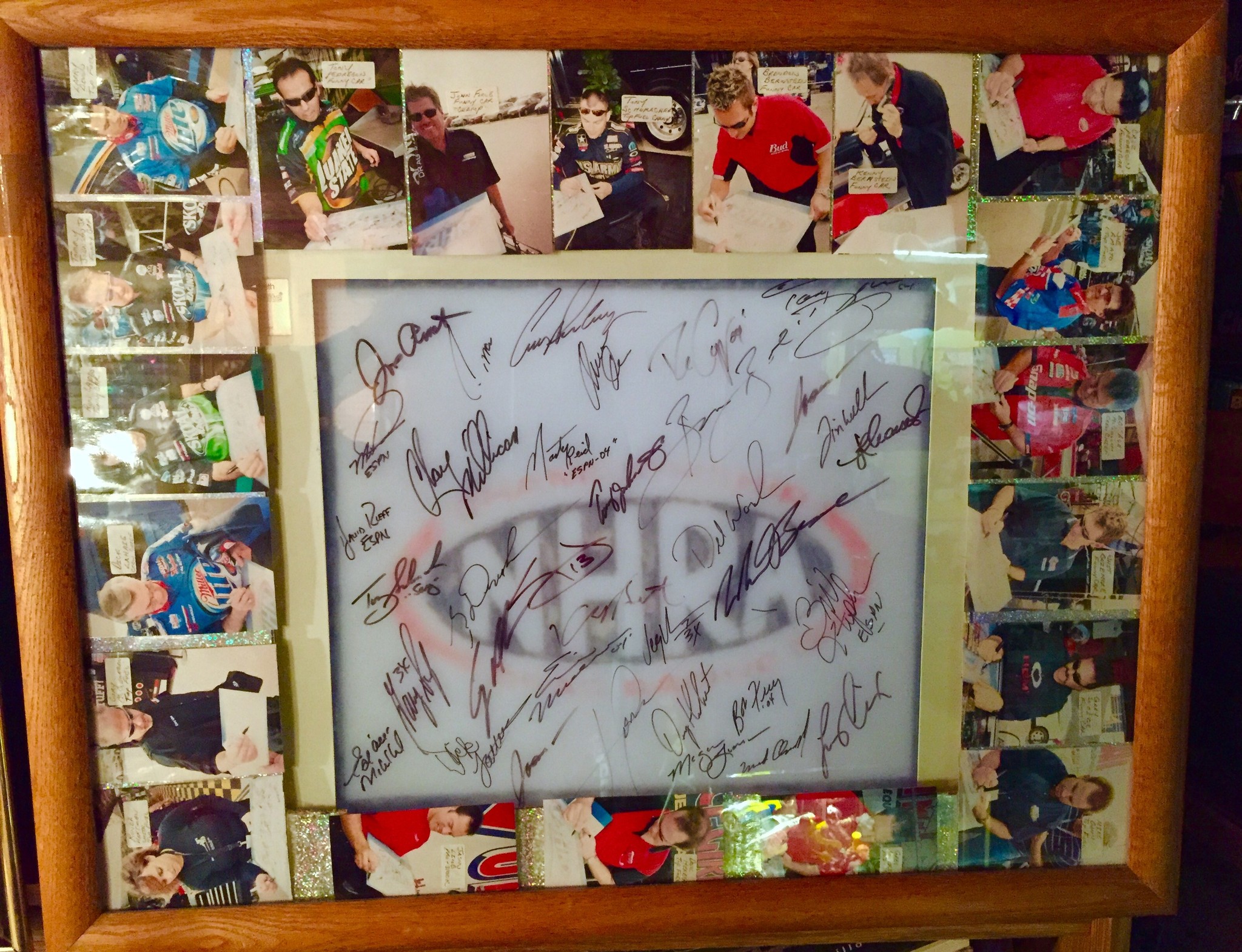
[[68, 50, 1159, 906]]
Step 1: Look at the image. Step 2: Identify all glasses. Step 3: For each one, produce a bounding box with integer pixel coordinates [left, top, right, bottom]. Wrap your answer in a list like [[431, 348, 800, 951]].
[[1079, 517, 1092, 541], [116, 707, 135, 744], [579, 108, 607, 116], [1072, 659, 1085, 687], [101, 271, 113, 305], [713, 114, 751, 129], [285, 84, 316, 107], [1139, 77, 1149, 115], [409, 106, 439, 122], [1104, 283, 1114, 310]]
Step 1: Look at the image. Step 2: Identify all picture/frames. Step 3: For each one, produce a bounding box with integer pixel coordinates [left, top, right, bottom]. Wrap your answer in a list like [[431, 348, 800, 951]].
[[0, 1, 1234, 952]]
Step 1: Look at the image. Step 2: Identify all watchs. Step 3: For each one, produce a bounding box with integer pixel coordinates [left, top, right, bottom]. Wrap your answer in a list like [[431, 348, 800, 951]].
[[815, 188, 831, 198], [1024, 247, 1039, 258], [998, 420, 1014, 431], [975, 815, 989, 824]]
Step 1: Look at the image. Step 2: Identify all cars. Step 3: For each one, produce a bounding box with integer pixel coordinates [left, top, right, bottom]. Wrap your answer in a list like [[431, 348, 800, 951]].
[[450, 94, 550, 128], [548, 50, 693, 152]]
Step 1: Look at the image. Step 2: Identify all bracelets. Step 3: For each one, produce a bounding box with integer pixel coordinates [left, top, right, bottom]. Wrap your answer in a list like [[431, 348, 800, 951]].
[[201, 381, 206, 391]]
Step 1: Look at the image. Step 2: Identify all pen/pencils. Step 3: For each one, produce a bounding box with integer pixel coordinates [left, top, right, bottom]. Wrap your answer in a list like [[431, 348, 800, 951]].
[[572, 829, 577, 836], [206, 124, 235, 144], [226, 584, 251, 604], [367, 871, 371, 879], [984, 512, 1008, 539], [313, 217, 331, 246], [204, 284, 224, 317], [250, 877, 276, 893], [989, 77, 1023, 109], [994, 377, 1019, 395], [572, 177, 586, 193], [766, 837, 788, 863], [226, 449, 259, 475], [977, 769, 1007, 793], [982, 640, 1006, 669], [1072, 223, 1080, 241], [242, 726, 249, 734], [710, 194, 718, 226]]
